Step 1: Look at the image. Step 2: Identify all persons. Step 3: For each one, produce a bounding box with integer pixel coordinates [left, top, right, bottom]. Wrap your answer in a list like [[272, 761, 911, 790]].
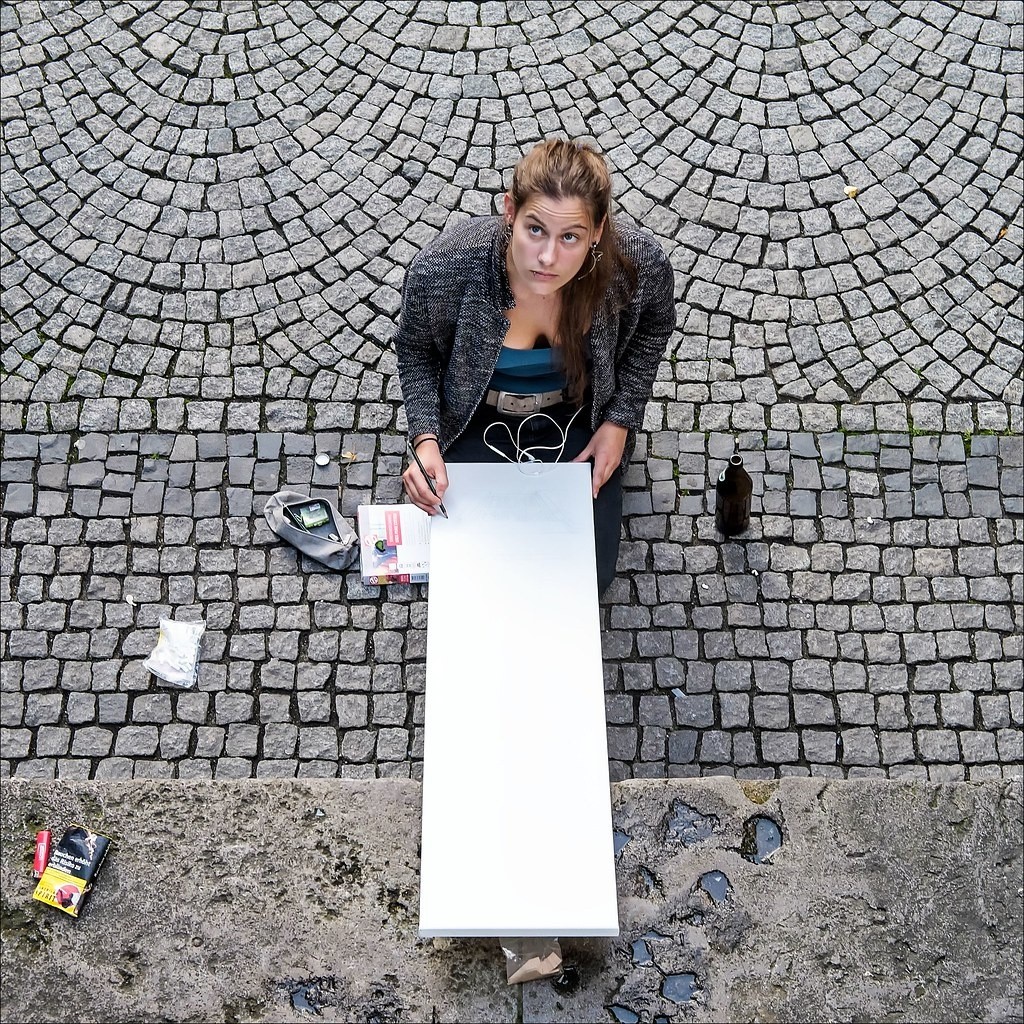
[[395, 138, 678, 603]]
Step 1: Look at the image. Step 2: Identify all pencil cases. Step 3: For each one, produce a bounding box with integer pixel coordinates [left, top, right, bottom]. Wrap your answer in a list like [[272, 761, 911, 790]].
[[264, 491, 361, 573]]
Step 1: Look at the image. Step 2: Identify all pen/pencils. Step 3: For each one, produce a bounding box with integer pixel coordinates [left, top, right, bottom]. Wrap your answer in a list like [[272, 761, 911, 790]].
[[283, 502, 307, 535], [404, 440, 454, 518]]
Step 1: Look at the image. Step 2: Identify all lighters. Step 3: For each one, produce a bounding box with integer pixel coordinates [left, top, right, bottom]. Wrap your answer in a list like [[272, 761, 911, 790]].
[[30, 831, 50, 879]]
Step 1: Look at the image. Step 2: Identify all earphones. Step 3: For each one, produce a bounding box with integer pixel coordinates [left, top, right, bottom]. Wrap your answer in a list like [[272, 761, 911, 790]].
[[520, 450, 535, 461]]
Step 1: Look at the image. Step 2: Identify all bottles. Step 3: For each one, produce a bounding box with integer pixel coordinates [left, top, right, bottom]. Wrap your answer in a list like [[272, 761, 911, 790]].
[[715, 454, 752, 536]]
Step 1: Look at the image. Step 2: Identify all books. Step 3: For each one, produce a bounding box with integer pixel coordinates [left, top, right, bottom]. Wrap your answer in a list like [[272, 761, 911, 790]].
[[30, 823, 113, 919], [355, 503, 430, 584]]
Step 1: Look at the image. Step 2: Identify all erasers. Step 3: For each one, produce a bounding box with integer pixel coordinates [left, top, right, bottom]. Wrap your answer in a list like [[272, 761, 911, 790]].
[[327, 532, 340, 543]]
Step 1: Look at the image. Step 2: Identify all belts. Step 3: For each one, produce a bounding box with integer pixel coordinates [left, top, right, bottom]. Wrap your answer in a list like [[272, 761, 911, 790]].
[[486, 389, 562, 417]]
